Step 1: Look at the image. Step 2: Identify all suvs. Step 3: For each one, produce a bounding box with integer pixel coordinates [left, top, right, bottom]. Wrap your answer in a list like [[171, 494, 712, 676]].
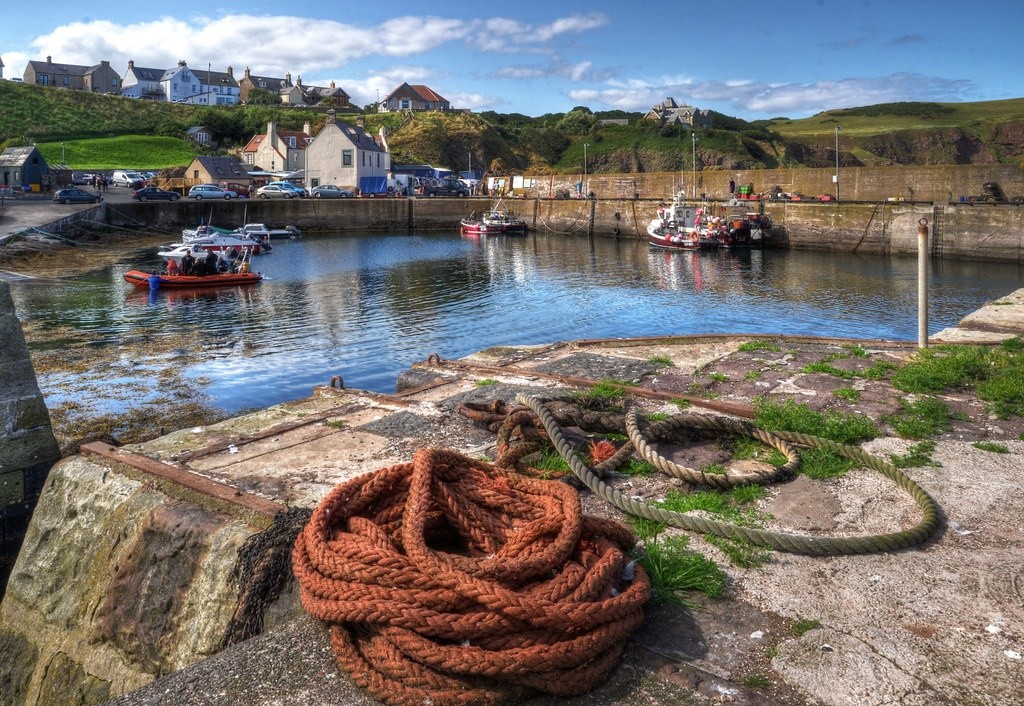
[[189, 184, 237, 200], [268, 181, 309, 198]]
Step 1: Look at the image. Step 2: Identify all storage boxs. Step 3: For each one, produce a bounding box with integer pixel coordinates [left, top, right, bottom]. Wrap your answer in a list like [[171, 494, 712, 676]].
[[748, 194, 760, 199], [737, 193, 750, 200], [821, 196, 833, 200], [791, 197, 801, 200], [737, 185, 751, 194]]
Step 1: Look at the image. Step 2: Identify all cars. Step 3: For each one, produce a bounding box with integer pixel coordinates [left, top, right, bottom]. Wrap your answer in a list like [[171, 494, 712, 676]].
[[256, 185, 298, 200], [72, 169, 161, 189], [53, 188, 105, 205], [386, 173, 472, 200], [132, 187, 182, 203], [309, 184, 354, 199]]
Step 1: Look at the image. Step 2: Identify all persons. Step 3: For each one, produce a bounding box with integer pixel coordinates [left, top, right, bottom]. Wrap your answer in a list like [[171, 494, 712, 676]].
[[92, 172, 110, 193], [248, 183, 256, 199], [152, 246, 242, 277], [729, 177, 737, 194]]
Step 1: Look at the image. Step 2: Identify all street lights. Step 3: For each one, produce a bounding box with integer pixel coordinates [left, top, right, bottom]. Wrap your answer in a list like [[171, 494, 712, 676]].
[[61, 143, 67, 165], [836, 125, 844, 203], [584, 142, 590, 199], [691, 132, 696, 200], [468, 151, 472, 197]]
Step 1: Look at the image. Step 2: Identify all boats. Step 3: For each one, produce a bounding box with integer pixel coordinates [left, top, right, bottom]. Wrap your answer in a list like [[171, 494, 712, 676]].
[[458, 194, 529, 238], [124, 203, 302, 290], [643, 187, 785, 254]]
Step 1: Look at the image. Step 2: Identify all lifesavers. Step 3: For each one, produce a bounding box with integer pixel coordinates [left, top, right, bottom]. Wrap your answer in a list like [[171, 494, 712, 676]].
[[615, 212, 620, 219], [690, 230, 699, 243], [613, 227, 619, 235]]
